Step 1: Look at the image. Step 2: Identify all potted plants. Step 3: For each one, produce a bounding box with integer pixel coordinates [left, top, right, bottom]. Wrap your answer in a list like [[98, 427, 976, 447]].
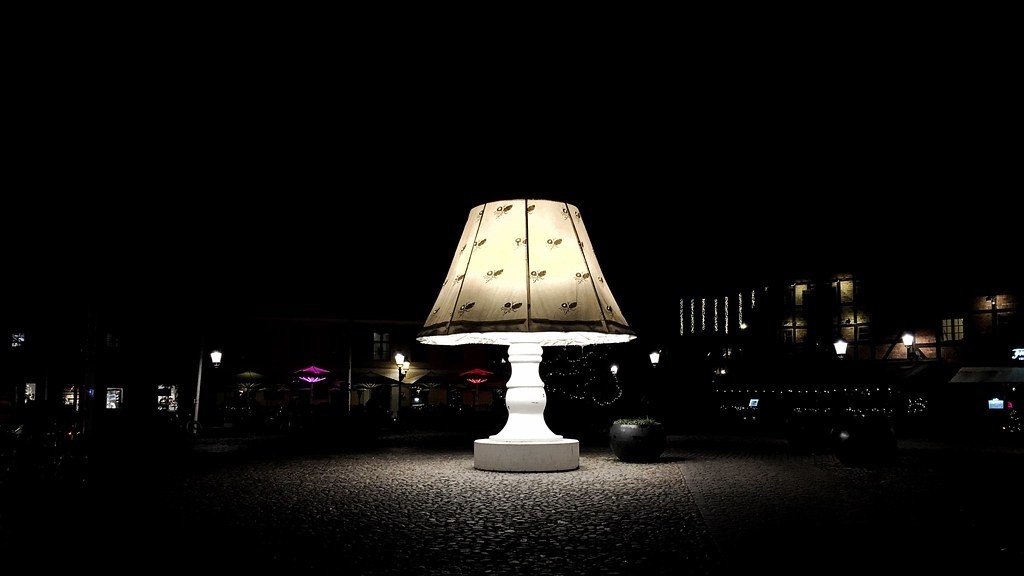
[[608, 414, 666, 463]]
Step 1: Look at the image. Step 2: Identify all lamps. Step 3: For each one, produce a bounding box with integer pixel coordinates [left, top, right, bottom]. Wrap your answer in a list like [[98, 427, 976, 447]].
[[415, 198, 637, 473]]
